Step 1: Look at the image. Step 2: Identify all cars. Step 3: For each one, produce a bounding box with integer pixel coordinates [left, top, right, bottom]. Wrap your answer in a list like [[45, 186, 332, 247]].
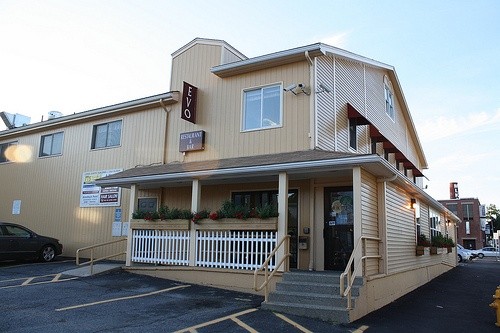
[[472, 247, 500, 258], [457, 243, 473, 263], [0, 222, 63, 263]]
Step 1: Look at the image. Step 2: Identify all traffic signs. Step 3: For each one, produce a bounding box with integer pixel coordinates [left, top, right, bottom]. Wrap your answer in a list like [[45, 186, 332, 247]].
[[488, 285, 500, 327]]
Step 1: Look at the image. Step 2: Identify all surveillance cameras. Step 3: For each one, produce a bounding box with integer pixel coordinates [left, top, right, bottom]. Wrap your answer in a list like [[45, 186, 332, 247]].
[[283, 84, 297, 92], [298, 83, 306, 88], [319, 84, 331, 93]]
